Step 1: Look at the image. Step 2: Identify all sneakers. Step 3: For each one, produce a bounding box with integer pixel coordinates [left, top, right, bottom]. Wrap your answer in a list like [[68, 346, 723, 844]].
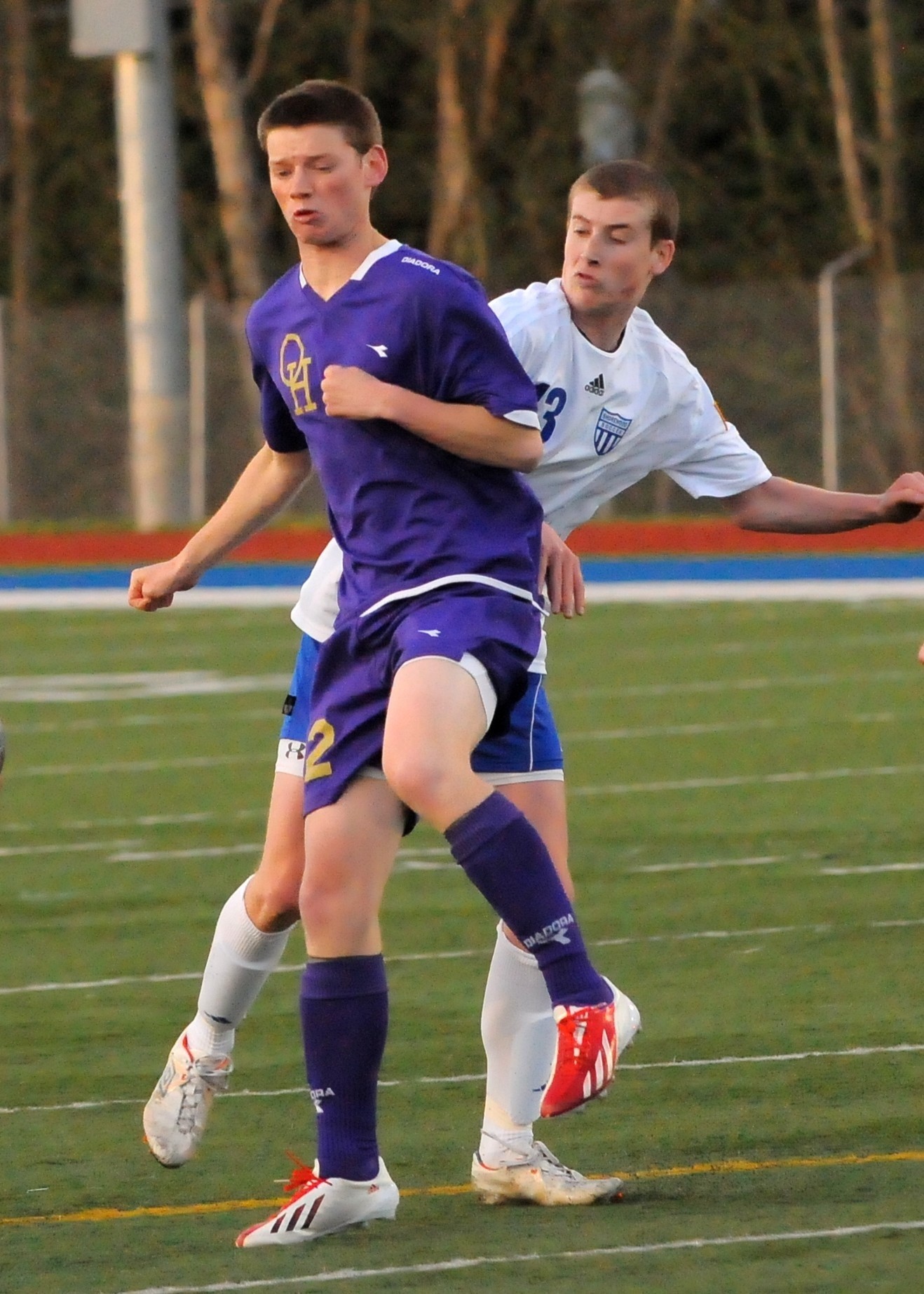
[[237, 1146, 400, 1250], [142, 1019, 234, 1169], [541, 973, 642, 1118], [472, 1141, 624, 1210]]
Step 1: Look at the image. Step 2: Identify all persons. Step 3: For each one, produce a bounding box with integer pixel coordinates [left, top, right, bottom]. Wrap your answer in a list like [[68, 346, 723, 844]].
[[140, 159, 923, 1219], [128, 78, 642, 1245]]
